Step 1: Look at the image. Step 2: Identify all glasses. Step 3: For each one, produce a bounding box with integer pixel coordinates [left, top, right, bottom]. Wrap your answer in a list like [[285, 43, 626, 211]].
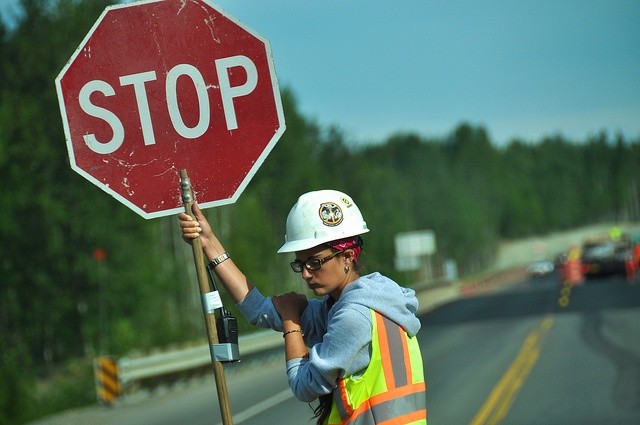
[[290, 241, 360, 273]]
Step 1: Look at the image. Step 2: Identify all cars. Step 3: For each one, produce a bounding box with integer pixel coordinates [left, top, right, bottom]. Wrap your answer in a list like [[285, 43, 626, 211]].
[[581, 241, 634, 283]]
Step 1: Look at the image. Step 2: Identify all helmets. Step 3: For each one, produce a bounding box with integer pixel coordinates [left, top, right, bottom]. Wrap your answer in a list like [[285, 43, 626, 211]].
[[276, 190, 370, 254]]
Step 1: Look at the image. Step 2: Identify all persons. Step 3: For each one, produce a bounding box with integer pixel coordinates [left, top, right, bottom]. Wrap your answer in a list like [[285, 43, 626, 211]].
[[178, 189, 428, 425]]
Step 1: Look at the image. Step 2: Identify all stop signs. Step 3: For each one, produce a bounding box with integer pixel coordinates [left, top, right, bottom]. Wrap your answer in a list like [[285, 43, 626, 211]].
[[53, 0, 287, 220]]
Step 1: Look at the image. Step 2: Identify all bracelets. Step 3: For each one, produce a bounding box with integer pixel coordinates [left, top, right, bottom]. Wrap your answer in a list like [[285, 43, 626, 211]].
[[283, 329, 304, 337], [209, 251, 231, 270]]
